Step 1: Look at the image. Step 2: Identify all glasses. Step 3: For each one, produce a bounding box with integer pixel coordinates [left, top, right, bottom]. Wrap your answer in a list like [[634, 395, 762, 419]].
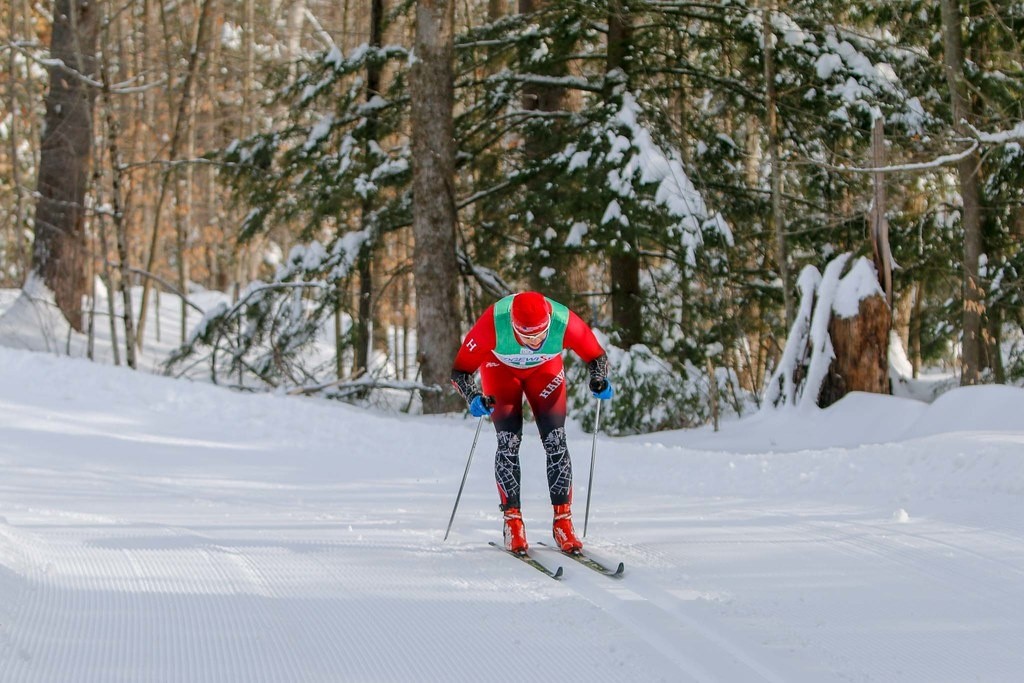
[[512, 314, 550, 340]]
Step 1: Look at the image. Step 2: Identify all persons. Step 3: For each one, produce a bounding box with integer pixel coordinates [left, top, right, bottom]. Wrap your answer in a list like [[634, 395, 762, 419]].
[[450, 291, 614, 558]]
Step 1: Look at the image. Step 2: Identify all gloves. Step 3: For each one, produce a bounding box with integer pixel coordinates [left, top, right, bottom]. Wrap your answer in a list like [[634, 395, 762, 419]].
[[469, 395, 494, 417], [592, 378, 613, 400]]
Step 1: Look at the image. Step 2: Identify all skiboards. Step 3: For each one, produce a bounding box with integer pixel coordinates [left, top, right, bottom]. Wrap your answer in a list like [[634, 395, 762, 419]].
[[488, 541, 625, 579]]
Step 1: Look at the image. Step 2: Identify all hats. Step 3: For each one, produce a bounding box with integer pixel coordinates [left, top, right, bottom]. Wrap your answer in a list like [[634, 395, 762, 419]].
[[511, 292, 549, 335]]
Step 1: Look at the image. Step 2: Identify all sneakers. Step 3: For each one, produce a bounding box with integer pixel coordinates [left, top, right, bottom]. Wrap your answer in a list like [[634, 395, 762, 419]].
[[553, 503, 583, 555], [503, 509, 528, 554]]
